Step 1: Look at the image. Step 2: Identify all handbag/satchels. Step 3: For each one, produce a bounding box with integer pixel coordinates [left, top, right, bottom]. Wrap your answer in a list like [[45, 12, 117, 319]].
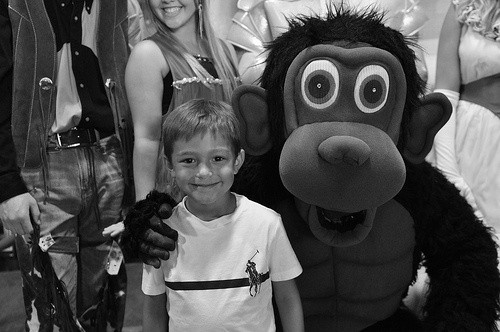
[[13, 214, 88, 332]]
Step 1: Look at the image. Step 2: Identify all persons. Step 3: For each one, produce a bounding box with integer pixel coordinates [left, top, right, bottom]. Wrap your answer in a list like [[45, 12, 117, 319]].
[[141, 97, 306, 332], [0, 1, 140, 332], [223, 0, 428, 94], [425, 0, 499, 273], [123, 0, 242, 206]]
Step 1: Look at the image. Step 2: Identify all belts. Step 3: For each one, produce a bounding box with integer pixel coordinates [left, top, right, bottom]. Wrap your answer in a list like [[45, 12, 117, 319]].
[[45, 124, 116, 150]]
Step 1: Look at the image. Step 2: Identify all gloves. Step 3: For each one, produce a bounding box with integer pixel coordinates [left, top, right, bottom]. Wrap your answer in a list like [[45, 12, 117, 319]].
[[431, 88, 478, 214]]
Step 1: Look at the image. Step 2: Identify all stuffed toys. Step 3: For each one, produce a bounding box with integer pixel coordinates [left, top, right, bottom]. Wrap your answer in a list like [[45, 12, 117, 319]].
[[119, 0, 500, 332]]
